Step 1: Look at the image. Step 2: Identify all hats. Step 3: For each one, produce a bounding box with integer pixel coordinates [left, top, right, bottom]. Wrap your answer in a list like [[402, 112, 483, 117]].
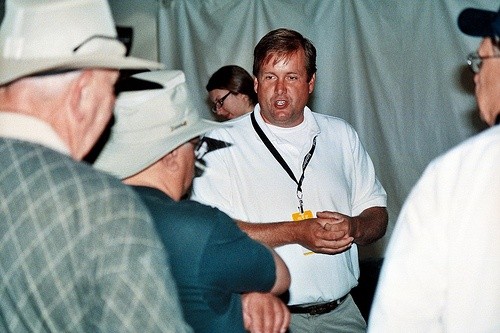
[[0, 0, 164, 86], [91, 69, 232, 181], [457, 7, 500, 38]]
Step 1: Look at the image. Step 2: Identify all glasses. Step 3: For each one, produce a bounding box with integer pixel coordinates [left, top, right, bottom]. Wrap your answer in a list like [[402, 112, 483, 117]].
[[467, 52, 500, 73], [73, 25, 133, 57], [210, 91, 231, 112]]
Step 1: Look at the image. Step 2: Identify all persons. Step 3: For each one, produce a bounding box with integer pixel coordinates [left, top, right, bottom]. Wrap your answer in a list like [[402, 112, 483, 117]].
[[367, 7, 500, 333], [0, 0, 193, 333], [180, 28, 390, 333], [79, 71, 291, 333], [205, 65, 258, 121]]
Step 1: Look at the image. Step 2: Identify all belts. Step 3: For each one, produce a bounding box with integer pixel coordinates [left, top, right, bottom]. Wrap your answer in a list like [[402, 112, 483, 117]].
[[287, 291, 348, 315]]
